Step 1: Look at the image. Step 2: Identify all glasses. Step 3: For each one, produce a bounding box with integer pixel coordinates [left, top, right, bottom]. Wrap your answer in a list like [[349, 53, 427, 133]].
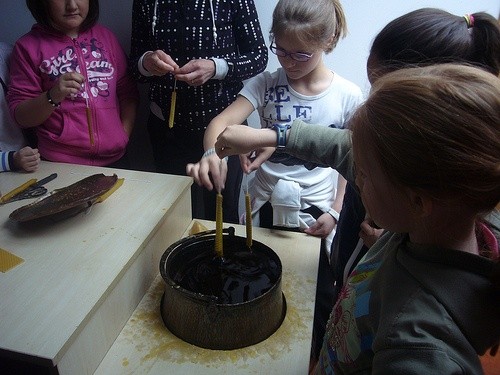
[[270, 37, 318, 62]]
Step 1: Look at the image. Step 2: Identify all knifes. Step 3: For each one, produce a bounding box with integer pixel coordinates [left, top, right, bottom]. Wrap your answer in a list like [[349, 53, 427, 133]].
[[0, 173, 57, 205]]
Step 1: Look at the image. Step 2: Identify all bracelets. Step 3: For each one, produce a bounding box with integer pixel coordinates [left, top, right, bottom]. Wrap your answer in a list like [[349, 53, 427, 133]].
[[200, 147, 231, 162], [326, 205, 341, 224], [47, 86, 62, 109]]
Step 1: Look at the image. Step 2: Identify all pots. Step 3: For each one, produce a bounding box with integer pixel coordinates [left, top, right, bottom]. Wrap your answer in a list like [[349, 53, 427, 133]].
[[160, 226, 284, 350]]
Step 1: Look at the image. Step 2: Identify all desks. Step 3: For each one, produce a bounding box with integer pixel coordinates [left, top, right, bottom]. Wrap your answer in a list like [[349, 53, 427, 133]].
[[0, 161, 322, 375]]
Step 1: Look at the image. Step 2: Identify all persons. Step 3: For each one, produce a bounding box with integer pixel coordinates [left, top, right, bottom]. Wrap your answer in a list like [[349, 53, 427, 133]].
[[239, 7, 500, 303], [187, 1, 365, 264], [6, 0, 135, 170], [130, 0, 268, 160], [216, 63, 500, 375], [0, 39, 41, 173]]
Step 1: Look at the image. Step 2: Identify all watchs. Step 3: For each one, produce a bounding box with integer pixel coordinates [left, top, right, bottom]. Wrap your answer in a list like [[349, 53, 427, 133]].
[[272, 119, 292, 149]]
